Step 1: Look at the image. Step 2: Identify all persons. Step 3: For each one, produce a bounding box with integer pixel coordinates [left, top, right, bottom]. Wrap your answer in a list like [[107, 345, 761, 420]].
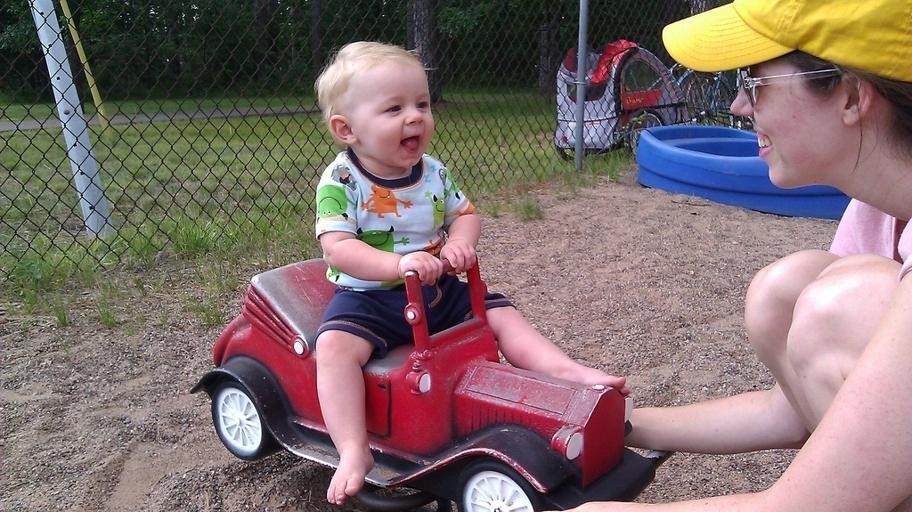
[[314, 40, 631, 506], [562, 0, 911, 511]]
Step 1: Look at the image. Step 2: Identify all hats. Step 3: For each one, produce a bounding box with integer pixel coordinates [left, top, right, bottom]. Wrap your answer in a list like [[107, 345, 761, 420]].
[[662, 0, 912, 84]]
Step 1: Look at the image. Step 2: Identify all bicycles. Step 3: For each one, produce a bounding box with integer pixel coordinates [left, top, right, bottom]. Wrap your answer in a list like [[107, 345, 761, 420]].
[[674, 68, 753, 131]]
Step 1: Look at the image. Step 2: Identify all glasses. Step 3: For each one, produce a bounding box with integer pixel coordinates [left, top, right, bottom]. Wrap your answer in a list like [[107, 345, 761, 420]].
[[738, 67, 842, 106]]
